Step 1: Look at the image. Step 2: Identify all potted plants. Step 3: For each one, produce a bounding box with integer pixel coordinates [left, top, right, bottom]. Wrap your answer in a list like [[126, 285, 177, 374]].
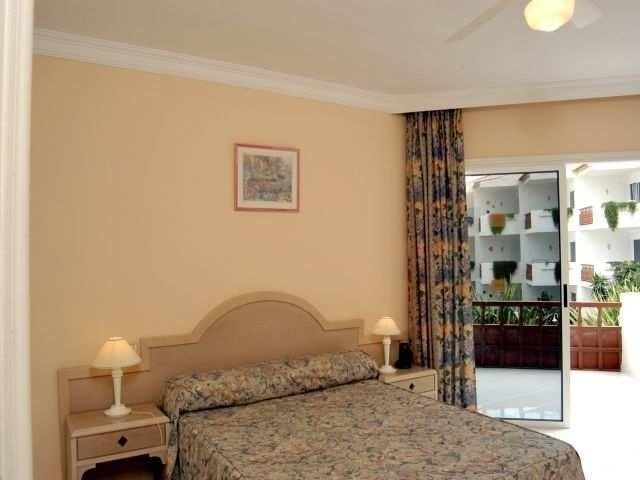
[[234, 142, 300, 213]]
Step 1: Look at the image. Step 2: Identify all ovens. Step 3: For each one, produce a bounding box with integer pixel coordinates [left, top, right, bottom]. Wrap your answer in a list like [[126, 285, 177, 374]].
[[158, 373, 582, 480]]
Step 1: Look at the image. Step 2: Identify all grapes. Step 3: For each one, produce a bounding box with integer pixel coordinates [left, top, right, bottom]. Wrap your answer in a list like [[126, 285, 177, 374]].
[[376, 363, 439, 400], [66, 399, 173, 478]]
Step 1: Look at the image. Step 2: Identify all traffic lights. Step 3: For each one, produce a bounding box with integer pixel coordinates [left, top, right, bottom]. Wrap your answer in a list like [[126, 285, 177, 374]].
[[161, 349, 381, 426]]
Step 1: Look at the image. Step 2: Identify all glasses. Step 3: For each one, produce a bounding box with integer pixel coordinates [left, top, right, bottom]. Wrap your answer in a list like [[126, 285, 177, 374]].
[[89, 338, 143, 417], [372, 316, 400, 375]]
